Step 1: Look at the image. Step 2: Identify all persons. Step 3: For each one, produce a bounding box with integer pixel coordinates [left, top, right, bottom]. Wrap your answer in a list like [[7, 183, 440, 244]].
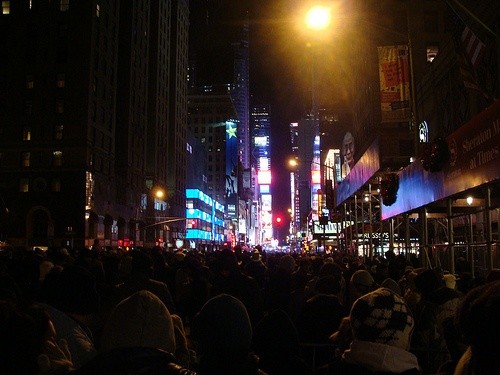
[[0, 216, 500, 375]]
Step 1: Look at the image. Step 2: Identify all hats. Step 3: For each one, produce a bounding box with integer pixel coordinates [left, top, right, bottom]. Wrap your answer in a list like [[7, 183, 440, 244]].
[[349, 287, 414, 343], [75, 248, 94, 257], [189, 294, 252, 358], [100, 289, 175, 353]]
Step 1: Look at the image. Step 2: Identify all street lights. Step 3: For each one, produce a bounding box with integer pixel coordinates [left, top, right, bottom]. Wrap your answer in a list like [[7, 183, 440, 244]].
[[133, 185, 166, 249], [142, 215, 196, 251], [287, 156, 341, 253]]
